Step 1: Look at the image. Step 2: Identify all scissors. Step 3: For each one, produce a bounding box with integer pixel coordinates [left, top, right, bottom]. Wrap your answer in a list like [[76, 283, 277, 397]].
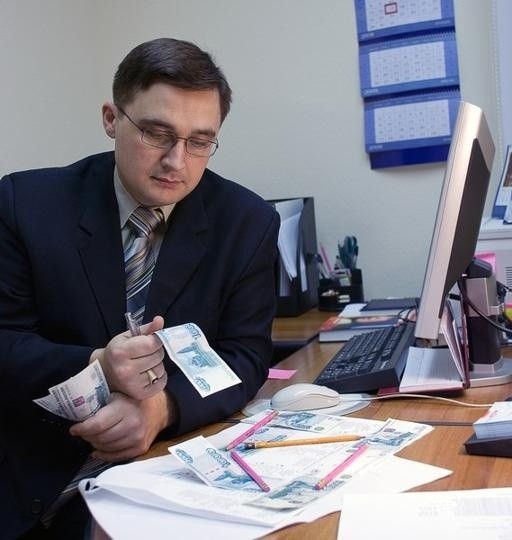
[[344, 236, 356, 269]]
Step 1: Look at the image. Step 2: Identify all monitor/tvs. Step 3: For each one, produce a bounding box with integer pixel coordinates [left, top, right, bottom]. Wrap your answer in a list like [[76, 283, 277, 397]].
[[414, 98, 512, 389]]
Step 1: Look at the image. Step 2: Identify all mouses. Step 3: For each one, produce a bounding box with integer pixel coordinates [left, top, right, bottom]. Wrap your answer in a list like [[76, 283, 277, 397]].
[[270, 382, 340, 411]]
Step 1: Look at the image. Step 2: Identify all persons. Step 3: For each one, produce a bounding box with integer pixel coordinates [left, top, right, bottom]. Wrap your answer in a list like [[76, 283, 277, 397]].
[[0, 36, 284, 539]]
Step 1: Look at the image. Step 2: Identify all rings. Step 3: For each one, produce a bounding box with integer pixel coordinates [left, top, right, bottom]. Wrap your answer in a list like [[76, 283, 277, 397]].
[[146, 368, 159, 385]]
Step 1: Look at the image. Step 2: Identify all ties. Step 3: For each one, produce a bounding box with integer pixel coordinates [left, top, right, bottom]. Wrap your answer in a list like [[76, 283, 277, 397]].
[[42, 206, 164, 529]]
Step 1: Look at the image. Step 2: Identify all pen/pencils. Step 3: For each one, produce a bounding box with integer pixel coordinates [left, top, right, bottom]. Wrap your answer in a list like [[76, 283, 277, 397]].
[[123, 313, 158, 387], [312, 239, 359, 280], [245, 435, 366, 449], [225, 410, 280, 451], [316, 444, 368, 489], [230, 452, 270, 492]]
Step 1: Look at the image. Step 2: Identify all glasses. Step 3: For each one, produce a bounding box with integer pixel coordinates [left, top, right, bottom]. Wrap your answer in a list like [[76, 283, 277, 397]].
[[122, 111, 219, 158]]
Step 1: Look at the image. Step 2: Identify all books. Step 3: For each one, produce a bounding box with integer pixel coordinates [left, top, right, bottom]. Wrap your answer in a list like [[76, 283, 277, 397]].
[[398, 294, 470, 394], [275, 197, 307, 297], [471, 399, 512, 440], [318, 298, 418, 342]]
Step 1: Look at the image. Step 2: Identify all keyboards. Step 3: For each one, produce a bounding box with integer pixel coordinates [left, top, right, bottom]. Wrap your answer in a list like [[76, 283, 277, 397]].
[[313, 323, 416, 393]]
